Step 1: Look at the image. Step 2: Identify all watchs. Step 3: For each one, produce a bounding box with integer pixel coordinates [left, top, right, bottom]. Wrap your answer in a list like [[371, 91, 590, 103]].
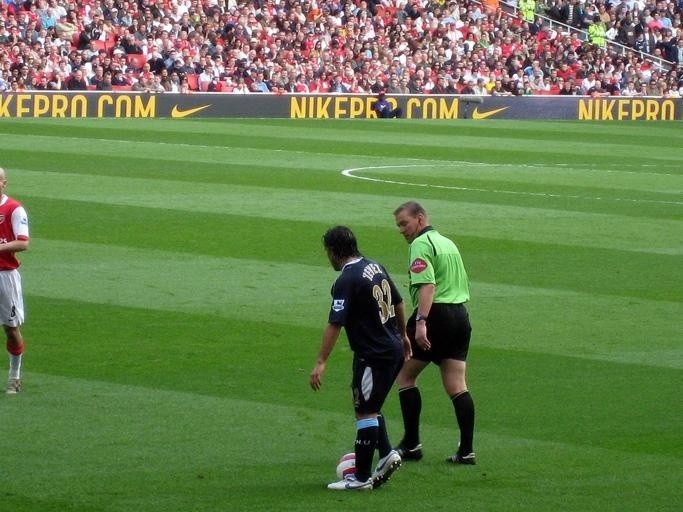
[[415, 313, 428, 321]]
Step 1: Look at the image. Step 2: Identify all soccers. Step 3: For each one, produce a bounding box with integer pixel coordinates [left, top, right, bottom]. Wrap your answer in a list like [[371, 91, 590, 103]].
[[336, 453, 358, 479]]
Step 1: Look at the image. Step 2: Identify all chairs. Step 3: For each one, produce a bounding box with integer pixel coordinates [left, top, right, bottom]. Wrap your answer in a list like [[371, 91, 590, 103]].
[[85, 28, 233, 92]]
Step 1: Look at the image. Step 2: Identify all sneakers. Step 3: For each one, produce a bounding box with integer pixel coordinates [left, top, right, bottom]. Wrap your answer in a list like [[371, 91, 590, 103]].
[[371, 449, 402, 488], [447, 452, 475, 464], [6, 379, 20, 394], [394, 443, 422, 461], [326, 472, 373, 490]]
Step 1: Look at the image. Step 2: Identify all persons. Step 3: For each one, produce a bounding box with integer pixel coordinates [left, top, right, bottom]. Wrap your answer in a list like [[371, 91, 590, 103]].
[[392, 201, 476, 463], [309, 227, 414, 489], [1, 0, 683, 118], [0, 170, 29, 394]]
